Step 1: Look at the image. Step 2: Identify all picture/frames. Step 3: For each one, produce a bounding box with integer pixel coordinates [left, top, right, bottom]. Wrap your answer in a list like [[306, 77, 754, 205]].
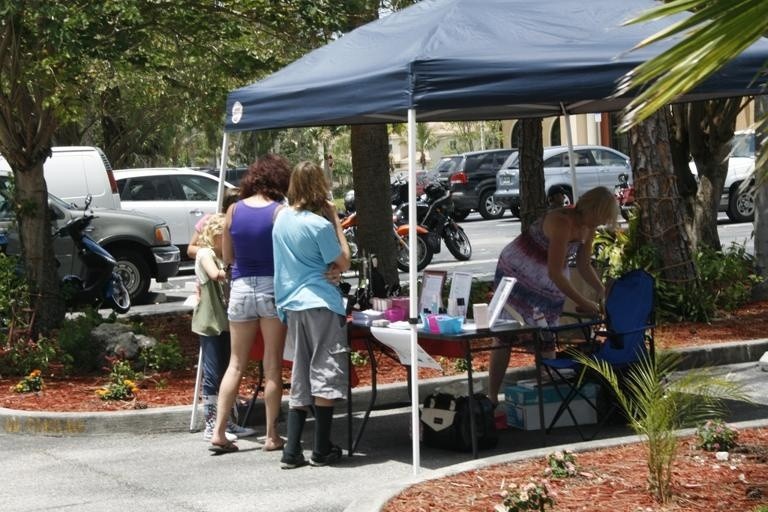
[[446, 269, 474, 323], [476, 276, 523, 329], [417, 269, 447, 314]]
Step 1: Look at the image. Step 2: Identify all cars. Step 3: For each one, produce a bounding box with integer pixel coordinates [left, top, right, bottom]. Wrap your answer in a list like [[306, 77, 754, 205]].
[[398, 170, 428, 197], [201, 167, 250, 189], [111, 168, 243, 274], [690, 129, 757, 223]]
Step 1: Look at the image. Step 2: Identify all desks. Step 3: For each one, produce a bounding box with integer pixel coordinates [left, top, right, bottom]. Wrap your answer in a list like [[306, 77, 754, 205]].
[[241, 296, 612, 461]]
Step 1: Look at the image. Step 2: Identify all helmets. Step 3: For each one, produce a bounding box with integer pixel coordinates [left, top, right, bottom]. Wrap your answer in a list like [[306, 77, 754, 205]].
[[424, 182, 445, 199], [344, 191, 355, 212]]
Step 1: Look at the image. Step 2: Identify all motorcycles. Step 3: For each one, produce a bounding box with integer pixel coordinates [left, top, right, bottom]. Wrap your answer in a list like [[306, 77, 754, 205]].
[[332, 172, 410, 223], [0, 196, 132, 314], [393, 169, 473, 260], [615, 173, 635, 222], [341, 210, 434, 272]]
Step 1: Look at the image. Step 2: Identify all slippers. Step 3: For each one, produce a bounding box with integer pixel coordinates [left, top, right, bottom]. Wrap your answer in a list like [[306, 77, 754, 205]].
[[262, 437, 288, 451], [208, 440, 238, 452]]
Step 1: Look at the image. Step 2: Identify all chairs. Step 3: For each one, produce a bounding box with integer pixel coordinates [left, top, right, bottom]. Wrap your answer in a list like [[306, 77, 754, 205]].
[[534, 269, 657, 436]]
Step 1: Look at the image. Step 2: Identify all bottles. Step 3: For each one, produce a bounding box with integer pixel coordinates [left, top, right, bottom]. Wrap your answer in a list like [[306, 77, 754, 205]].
[[531, 307, 555, 342]]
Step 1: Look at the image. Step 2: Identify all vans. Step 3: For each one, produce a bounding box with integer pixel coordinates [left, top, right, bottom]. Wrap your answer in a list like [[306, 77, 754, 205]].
[[0, 145, 123, 212]]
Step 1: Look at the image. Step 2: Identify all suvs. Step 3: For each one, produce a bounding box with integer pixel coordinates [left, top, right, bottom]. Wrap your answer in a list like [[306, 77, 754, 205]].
[[492, 146, 633, 214], [436, 147, 521, 222], [0, 170, 178, 305]]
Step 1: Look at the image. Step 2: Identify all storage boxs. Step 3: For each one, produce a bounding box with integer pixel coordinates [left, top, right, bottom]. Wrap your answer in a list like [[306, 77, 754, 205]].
[[502, 383, 599, 431]]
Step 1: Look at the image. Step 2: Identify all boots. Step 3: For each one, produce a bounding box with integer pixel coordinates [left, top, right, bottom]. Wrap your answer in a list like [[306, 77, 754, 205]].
[[202, 394, 257, 442]]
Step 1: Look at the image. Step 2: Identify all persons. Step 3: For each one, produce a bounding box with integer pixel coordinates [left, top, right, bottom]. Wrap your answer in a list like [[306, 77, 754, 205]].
[[207, 155, 291, 452], [546, 186, 565, 209], [191, 213, 256, 442], [486, 186, 619, 406], [271, 160, 351, 470], [186, 189, 243, 260]]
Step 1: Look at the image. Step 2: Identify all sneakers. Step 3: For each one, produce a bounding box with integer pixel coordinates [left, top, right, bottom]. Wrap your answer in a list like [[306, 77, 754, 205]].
[[279, 451, 305, 467], [309, 442, 342, 465]]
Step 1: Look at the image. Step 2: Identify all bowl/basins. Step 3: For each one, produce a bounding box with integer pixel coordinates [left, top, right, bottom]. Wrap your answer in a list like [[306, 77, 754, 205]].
[[419, 312, 465, 335]]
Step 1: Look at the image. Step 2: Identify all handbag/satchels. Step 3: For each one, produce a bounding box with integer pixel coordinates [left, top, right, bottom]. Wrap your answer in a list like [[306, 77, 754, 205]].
[[421, 392, 495, 452]]
